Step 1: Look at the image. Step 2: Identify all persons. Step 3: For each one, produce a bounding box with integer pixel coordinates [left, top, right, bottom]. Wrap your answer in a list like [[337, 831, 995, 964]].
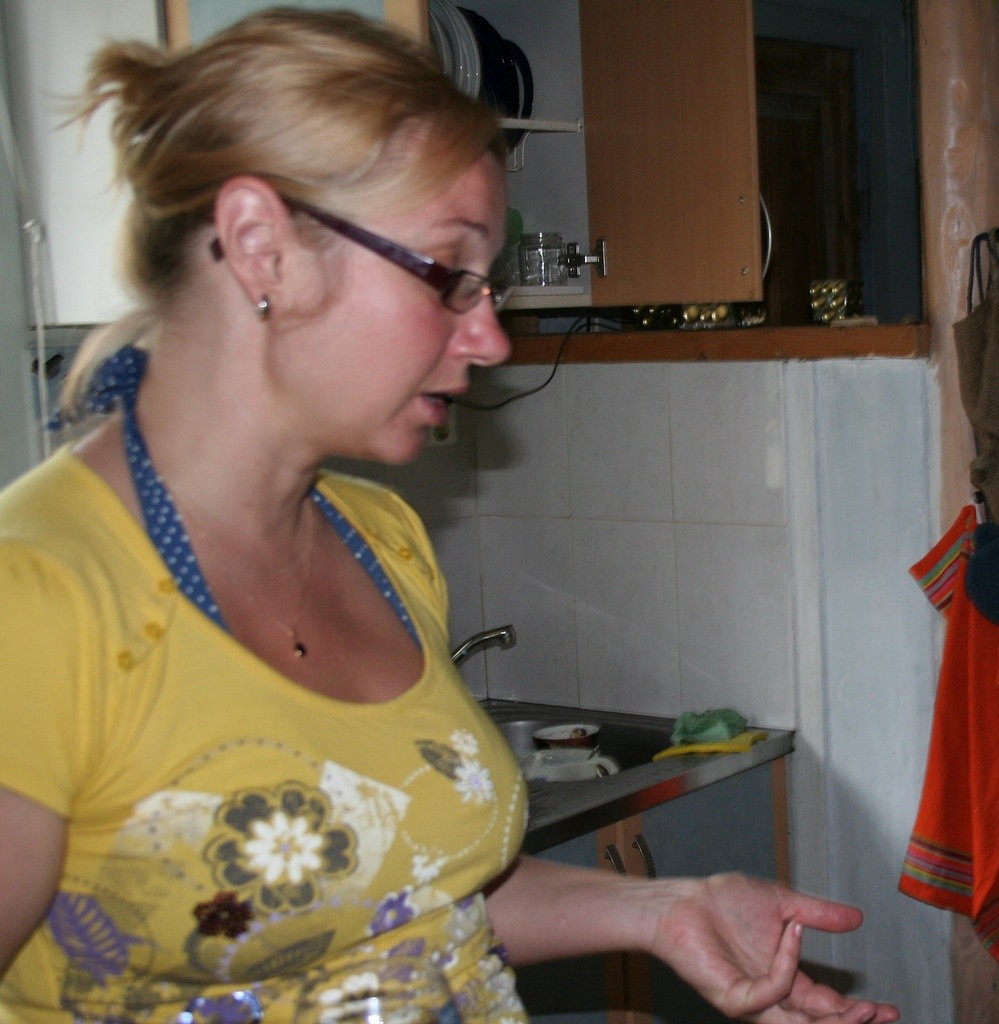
[[0, 7, 902, 1024]]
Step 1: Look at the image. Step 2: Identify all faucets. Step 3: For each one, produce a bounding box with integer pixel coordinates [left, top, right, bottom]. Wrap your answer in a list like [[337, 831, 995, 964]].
[[450, 624, 518, 669]]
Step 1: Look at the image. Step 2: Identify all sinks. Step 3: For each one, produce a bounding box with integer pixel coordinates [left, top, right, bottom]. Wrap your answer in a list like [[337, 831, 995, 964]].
[[484, 697, 694, 786]]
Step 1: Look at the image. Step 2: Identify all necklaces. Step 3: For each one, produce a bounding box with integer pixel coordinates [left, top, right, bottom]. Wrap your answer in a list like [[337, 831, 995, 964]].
[[170, 482, 319, 659]]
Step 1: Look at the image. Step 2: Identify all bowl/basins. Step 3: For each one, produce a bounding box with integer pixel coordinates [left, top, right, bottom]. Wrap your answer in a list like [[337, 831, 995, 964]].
[[531, 723, 600, 750]]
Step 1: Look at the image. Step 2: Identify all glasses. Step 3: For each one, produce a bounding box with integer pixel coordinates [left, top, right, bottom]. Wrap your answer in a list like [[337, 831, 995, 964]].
[[208, 194, 514, 316]]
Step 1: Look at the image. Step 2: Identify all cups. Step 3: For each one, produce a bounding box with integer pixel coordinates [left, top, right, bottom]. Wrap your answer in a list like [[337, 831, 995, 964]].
[[533, 749, 620, 783], [518, 232, 567, 287]]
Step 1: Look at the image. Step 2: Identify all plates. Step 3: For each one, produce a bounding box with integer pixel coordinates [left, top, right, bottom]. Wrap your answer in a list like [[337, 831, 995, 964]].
[[426, 0, 533, 149]]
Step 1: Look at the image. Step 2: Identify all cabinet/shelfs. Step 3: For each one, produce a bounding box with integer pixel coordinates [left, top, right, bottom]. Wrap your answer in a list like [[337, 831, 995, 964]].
[[519, 755, 791, 1023], [2, 0, 773, 326]]
